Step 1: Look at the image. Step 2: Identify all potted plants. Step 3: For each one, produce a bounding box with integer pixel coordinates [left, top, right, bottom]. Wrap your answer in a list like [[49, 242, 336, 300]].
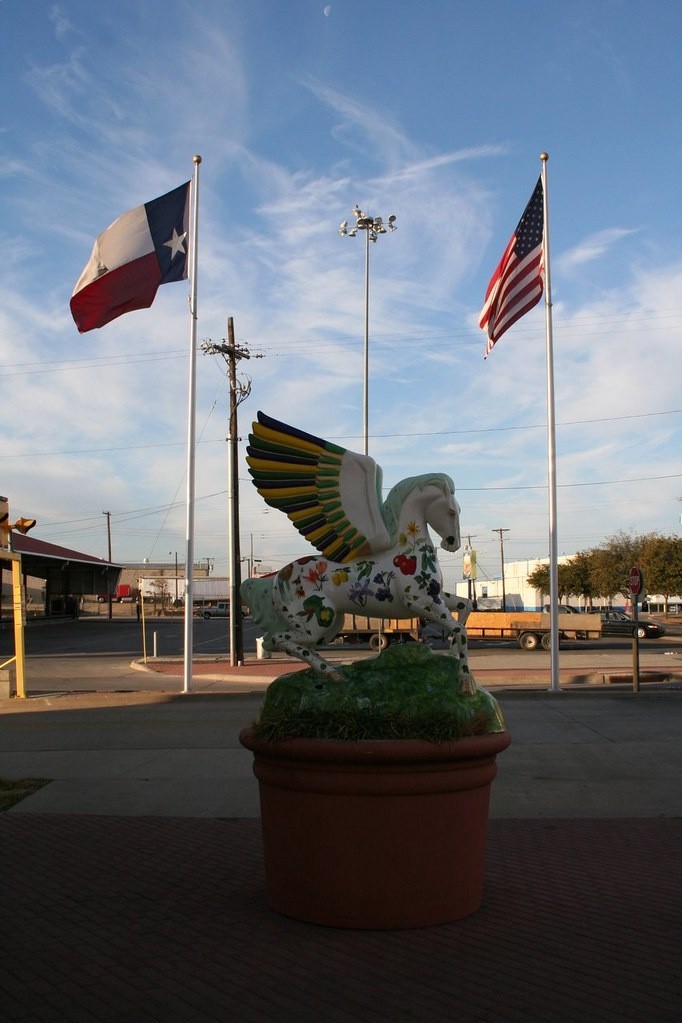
[[238, 640, 512, 930]]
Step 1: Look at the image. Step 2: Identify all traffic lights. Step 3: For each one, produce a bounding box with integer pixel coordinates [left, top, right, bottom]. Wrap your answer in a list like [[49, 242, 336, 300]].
[[13, 516, 36, 534]]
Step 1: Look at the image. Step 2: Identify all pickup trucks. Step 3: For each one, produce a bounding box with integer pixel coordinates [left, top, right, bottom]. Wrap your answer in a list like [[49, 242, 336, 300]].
[[196, 600, 249, 619]]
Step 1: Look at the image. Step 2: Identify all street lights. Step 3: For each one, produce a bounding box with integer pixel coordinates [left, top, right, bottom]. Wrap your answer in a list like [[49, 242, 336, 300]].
[[240, 557, 251, 579], [338, 203, 397, 456]]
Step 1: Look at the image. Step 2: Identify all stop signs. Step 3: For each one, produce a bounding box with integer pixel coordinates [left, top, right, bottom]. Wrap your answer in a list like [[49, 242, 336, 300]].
[[628, 567, 642, 595]]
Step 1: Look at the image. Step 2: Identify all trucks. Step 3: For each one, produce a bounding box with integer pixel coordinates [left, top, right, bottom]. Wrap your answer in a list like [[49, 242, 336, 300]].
[[172, 577, 230, 608]]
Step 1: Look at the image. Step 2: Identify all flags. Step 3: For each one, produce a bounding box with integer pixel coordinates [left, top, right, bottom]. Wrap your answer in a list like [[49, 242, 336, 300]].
[[478, 171, 544, 359], [70, 178, 191, 334]]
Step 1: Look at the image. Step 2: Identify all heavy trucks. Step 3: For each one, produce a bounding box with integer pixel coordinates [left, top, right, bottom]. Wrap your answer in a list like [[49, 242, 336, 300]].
[[98, 574, 189, 604]]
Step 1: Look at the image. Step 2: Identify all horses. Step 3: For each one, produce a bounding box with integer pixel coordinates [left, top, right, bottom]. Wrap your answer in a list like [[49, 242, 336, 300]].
[[239, 473, 478, 697]]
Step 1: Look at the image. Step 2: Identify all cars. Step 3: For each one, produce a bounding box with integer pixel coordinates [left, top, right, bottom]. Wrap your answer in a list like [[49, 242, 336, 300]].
[[543, 604, 580, 614], [589, 610, 666, 639]]
